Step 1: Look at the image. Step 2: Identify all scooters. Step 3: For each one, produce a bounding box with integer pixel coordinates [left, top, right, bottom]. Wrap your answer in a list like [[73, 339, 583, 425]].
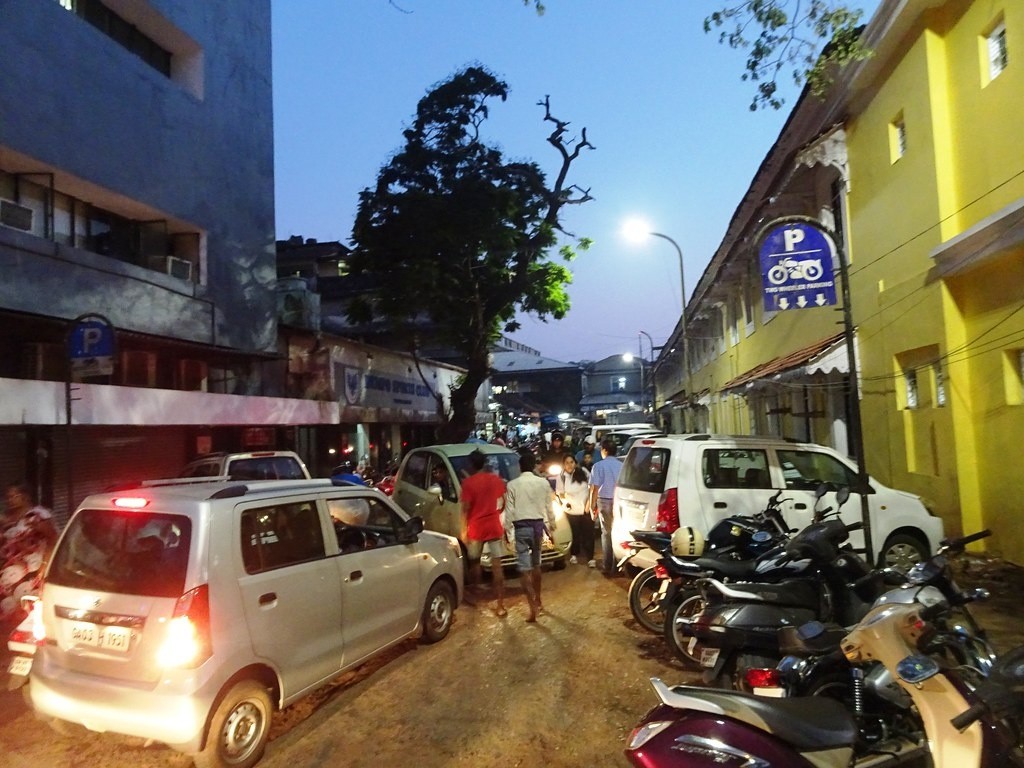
[[616, 480, 1024, 768], [346, 452, 400, 483]]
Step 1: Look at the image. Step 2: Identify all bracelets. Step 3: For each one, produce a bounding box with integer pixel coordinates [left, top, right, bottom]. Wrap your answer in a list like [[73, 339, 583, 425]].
[[41, 561, 48, 564]]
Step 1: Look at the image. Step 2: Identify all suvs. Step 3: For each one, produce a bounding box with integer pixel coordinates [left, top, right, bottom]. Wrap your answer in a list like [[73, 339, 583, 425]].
[[31, 476, 464, 768]]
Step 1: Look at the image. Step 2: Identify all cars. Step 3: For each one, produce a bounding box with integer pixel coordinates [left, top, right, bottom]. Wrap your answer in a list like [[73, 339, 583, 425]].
[[611, 432, 944, 577], [578, 422, 669, 476], [395, 443, 572, 573]]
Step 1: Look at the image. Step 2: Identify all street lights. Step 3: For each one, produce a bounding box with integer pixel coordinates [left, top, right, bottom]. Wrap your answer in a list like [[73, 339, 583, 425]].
[[619, 215, 697, 435], [623, 353, 645, 410]]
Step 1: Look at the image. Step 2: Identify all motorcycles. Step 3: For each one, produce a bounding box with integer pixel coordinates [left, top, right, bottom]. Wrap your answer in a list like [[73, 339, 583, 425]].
[[6, 592, 41, 711]]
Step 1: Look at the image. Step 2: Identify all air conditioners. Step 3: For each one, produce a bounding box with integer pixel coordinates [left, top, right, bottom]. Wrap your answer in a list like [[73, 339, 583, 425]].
[[0, 196, 36, 235], [151, 255, 193, 282]]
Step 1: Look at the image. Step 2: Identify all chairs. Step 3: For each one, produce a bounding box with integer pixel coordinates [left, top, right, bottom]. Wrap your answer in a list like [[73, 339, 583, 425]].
[[745, 469, 769, 488], [294, 510, 322, 556]]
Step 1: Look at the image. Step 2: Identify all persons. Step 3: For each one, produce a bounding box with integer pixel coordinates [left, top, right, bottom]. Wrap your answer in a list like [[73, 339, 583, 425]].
[[501, 451, 556, 621], [0, 480, 58, 591], [457, 448, 508, 616], [552, 435, 625, 577], [466, 425, 518, 447]]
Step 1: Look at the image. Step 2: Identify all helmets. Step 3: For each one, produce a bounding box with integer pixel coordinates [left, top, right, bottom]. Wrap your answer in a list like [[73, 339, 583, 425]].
[[552, 430, 563, 442], [584, 435, 596, 444], [672, 526, 704, 558]]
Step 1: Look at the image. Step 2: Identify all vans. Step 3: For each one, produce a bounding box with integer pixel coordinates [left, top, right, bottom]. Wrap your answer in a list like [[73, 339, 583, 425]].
[[182, 449, 313, 482]]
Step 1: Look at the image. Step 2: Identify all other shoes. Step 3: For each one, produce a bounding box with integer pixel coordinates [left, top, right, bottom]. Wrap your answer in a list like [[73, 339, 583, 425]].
[[587, 559, 596, 568], [569, 555, 579, 564]]
